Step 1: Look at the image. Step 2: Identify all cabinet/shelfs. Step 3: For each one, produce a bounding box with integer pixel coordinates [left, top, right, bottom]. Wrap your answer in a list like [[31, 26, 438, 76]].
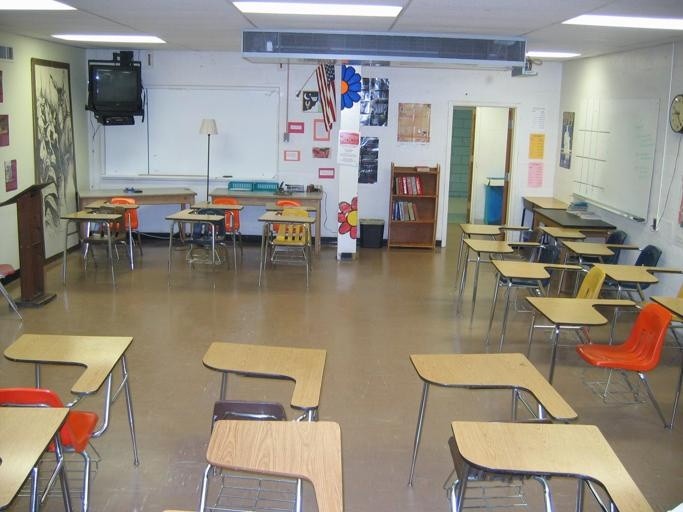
[[387, 162, 440, 251]]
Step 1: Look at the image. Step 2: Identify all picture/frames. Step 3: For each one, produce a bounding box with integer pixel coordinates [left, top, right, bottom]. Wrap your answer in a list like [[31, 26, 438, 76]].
[[28, 55, 79, 231]]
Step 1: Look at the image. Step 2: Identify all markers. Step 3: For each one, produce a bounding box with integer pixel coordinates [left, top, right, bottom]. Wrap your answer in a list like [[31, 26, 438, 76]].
[[223, 176, 233, 177]]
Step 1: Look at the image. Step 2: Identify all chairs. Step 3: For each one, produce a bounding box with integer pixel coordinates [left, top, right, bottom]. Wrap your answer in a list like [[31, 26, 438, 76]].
[[207, 401, 289, 437], [0, 385, 104, 500], [440, 416, 552, 509]]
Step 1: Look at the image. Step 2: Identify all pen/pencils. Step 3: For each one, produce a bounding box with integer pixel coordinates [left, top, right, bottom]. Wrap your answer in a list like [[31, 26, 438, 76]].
[[280, 181, 285, 188]]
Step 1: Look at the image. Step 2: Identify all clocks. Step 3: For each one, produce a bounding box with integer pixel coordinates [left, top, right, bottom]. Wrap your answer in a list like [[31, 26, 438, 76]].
[[666, 94, 682, 137]]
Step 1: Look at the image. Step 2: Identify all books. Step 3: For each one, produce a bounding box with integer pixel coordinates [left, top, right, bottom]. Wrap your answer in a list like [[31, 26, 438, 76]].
[[393, 175, 423, 222]]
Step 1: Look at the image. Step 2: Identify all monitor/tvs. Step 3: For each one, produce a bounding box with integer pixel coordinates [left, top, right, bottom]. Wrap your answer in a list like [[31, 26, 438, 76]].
[[85, 65, 143, 112]]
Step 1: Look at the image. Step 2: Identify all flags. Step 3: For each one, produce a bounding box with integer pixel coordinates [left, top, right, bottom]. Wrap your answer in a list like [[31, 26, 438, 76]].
[[315, 63, 336, 132]]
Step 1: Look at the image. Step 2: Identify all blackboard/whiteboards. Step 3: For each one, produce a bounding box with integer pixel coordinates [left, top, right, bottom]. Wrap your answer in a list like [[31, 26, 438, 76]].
[[100, 83, 280, 183], [569, 97, 660, 224]]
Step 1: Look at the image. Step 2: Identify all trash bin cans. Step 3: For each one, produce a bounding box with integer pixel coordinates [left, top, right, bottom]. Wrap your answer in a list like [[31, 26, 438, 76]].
[[359, 219, 385, 248]]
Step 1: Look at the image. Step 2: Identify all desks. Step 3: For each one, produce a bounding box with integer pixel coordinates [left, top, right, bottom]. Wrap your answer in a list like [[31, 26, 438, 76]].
[[0, 401, 73, 512], [198, 340, 330, 423], [447, 421, 660, 512], [60, 186, 324, 287], [3, 330, 144, 470], [198, 418, 347, 512], [405, 351, 581, 489]]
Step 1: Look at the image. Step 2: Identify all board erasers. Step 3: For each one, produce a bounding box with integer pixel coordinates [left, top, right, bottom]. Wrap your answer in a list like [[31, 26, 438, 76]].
[[633, 217, 645, 222]]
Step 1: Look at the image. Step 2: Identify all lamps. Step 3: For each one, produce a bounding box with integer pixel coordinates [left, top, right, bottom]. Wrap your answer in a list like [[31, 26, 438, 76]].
[[196, 118, 220, 208]]
